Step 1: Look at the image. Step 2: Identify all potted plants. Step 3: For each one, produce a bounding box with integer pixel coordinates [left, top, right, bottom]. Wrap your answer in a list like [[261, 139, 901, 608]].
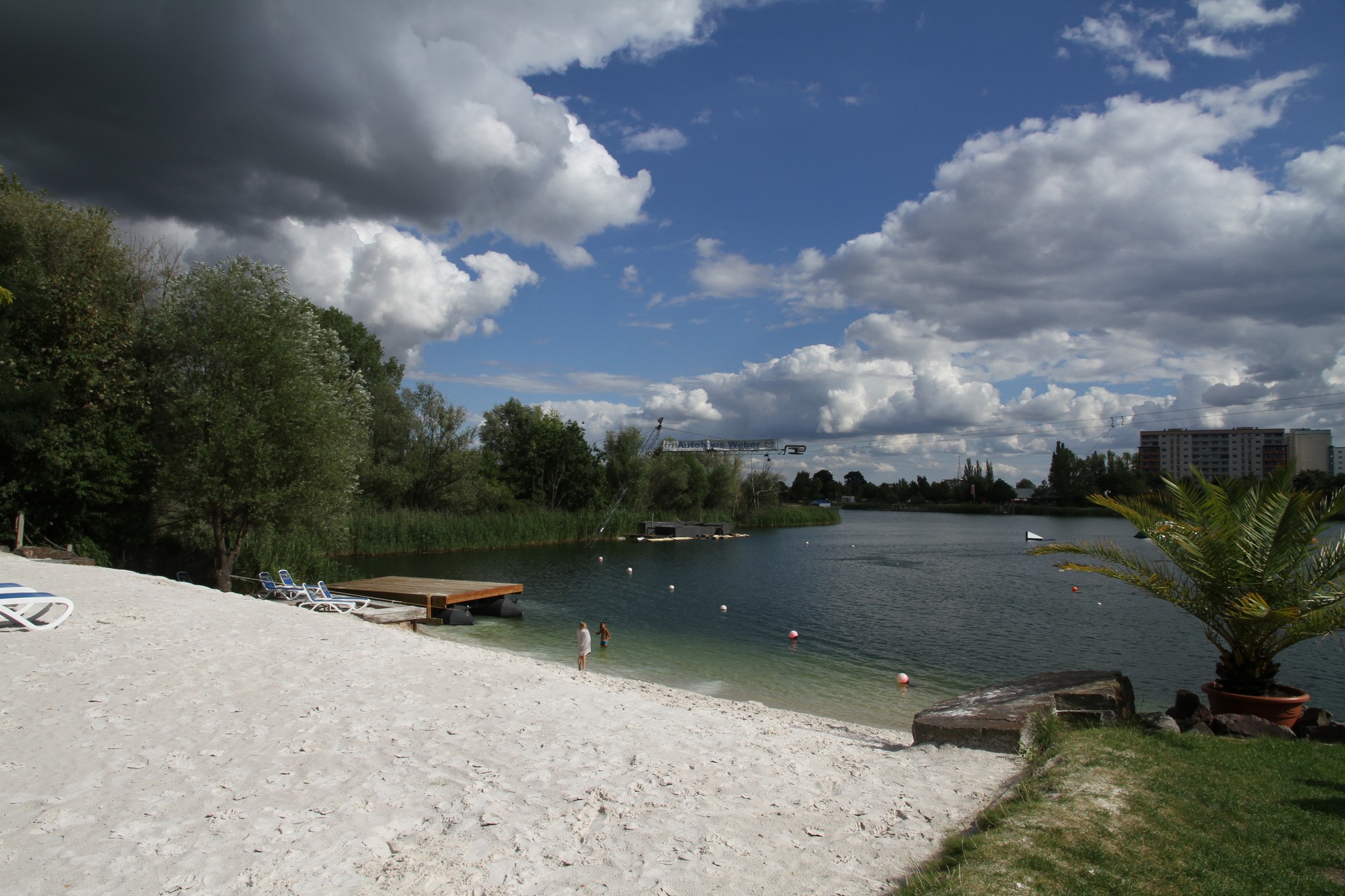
[[1024, 462, 1345, 729]]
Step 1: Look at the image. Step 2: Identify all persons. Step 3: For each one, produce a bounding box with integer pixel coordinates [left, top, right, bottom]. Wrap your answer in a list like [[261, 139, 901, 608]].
[[577, 621, 591, 671], [594, 622, 610, 647]]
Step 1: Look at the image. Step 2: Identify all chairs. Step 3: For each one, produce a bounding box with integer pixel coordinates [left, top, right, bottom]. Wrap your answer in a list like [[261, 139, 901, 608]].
[[0, 582, 36, 614], [0, 592, 74, 630], [257, 572, 306, 601], [315, 581, 371, 611], [298, 583, 356, 614], [276, 570, 322, 596], [177, 571, 193, 584]]
